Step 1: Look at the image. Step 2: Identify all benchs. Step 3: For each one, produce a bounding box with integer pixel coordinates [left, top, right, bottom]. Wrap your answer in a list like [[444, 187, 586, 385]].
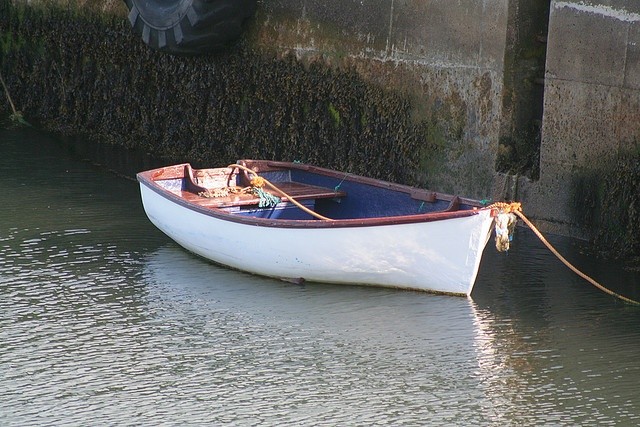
[[170, 181, 347, 219]]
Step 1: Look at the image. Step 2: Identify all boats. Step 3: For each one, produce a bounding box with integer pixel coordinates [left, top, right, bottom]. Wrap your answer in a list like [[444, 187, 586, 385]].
[[137, 159, 498, 298]]
